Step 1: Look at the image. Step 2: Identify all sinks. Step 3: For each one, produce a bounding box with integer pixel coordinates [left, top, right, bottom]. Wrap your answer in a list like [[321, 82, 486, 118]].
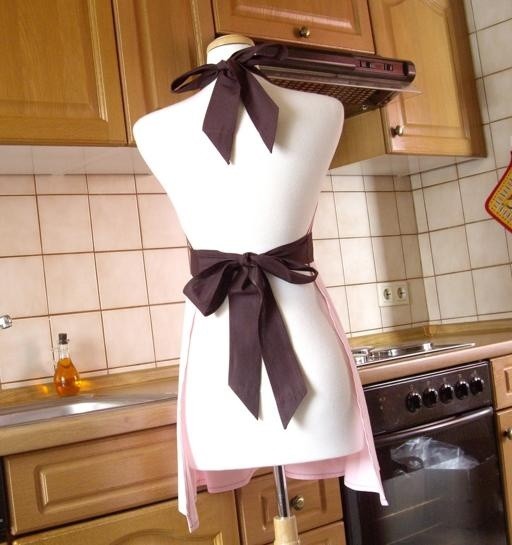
[[1, 392, 177, 430]]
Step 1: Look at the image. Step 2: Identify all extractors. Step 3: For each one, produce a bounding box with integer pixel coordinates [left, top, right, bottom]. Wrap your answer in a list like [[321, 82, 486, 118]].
[[253, 41, 418, 120]]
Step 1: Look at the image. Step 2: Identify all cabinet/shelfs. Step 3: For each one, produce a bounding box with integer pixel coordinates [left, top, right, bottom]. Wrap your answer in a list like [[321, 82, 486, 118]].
[[236, 472, 347, 545], [329, 0, 489, 170], [4, 425, 243, 545], [211, 0, 376, 56]]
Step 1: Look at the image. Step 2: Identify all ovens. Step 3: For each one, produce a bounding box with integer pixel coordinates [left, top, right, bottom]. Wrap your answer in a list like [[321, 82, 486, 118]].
[[339, 359, 510, 544]]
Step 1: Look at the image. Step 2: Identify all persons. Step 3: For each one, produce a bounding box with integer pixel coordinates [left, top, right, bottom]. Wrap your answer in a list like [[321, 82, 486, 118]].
[[130, 32, 364, 472]]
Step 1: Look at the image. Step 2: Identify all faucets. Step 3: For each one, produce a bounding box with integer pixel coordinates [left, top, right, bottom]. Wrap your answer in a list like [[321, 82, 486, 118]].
[[1, 312, 14, 332]]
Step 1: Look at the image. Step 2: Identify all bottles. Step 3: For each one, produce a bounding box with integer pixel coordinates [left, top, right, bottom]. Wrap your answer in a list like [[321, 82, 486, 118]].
[[53, 332, 81, 398]]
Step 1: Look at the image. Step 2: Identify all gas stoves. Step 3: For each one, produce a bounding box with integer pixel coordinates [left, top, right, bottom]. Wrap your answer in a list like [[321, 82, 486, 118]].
[[344, 340, 476, 372]]
